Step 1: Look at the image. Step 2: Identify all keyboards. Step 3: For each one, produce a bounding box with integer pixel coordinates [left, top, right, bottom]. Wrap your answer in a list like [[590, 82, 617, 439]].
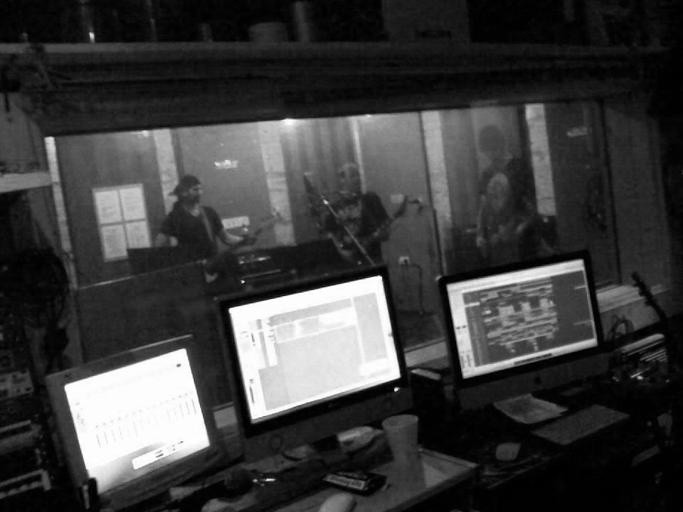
[[531, 405, 628, 445]]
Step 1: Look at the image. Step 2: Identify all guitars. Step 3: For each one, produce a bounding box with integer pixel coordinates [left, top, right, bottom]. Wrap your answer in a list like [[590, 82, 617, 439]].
[[346, 194, 424, 262], [227, 213, 294, 252]]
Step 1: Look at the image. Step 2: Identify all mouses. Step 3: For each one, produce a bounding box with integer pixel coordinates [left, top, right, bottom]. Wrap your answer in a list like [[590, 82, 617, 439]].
[[315, 495, 354, 512]]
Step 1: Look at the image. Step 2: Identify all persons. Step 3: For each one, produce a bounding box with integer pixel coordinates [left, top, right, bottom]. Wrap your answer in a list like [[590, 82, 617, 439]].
[[321, 161, 389, 266], [473, 124, 536, 267], [153, 176, 256, 264]]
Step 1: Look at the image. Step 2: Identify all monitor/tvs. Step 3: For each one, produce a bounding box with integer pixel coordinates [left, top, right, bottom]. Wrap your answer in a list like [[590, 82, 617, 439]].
[[214, 263, 411, 466], [437, 249, 607, 426], [43, 334, 224, 512]]
